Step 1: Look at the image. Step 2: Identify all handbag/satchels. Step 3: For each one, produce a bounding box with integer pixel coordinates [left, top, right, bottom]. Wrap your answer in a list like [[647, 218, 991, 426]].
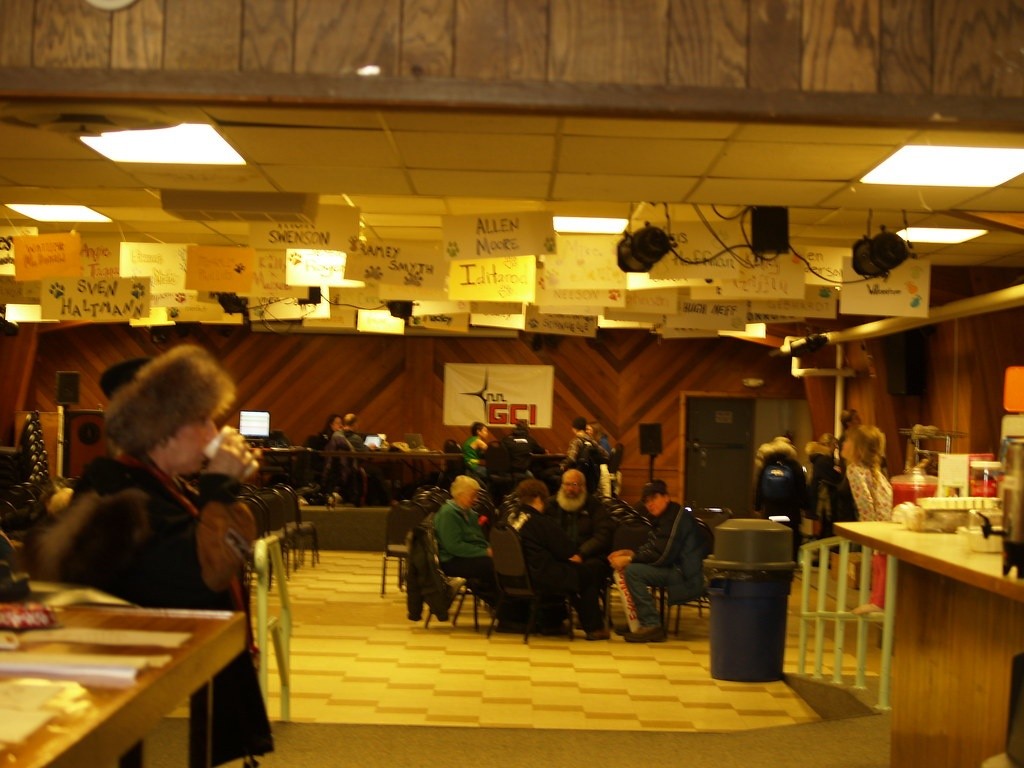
[[693, 517, 715, 558]]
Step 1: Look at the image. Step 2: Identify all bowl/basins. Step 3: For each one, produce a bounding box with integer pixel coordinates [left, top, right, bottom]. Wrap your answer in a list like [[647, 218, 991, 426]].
[[925, 509, 968, 533]]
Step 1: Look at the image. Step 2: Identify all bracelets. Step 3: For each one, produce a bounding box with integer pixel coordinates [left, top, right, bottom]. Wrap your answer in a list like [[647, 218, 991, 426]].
[[628, 556, 632, 561]]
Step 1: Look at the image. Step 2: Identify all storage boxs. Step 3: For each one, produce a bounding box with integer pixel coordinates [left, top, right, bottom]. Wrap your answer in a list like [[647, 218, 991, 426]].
[[956, 525, 1004, 553], [831, 552, 839, 582], [848, 552, 874, 590]]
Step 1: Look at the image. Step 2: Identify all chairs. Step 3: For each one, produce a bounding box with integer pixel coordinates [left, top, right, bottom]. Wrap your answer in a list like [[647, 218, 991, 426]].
[[235, 480, 320, 590], [380, 499, 731, 643]]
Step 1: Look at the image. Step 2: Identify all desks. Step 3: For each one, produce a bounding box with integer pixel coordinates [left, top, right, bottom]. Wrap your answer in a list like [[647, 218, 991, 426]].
[[0, 600, 246, 768], [260, 444, 587, 508]]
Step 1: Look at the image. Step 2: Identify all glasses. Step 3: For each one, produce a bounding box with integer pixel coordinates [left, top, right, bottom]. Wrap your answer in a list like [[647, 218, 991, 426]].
[[563, 481, 581, 487]]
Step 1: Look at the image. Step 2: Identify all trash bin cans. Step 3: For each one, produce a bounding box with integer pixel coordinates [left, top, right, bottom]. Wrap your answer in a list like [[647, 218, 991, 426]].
[[700, 518, 796, 682]]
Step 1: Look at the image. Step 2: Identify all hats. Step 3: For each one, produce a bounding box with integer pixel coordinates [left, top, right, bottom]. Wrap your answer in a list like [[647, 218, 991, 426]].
[[643, 479, 666, 494], [106, 345, 232, 456], [572, 417, 586, 429]]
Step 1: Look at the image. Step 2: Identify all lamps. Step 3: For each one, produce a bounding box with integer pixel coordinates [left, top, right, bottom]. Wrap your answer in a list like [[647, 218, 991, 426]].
[[214, 290, 250, 315], [384, 298, 416, 322], [617, 198, 670, 275], [851, 208, 913, 283]]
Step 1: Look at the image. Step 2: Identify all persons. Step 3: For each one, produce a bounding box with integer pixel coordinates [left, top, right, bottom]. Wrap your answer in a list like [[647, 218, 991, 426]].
[[841, 425, 893, 614], [435, 470, 708, 643], [754, 432, 806, 570], [313, 412, 387, 498], [802, 433, 840, 567], [42, 345, 274, 768], [465, 416, 610, 490], [838, 409, 888, 552]]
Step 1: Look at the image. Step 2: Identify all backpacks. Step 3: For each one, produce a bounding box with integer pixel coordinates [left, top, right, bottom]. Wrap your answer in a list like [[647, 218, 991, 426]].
[[576, 436, 600, 464], [762, 460, 794, 499]]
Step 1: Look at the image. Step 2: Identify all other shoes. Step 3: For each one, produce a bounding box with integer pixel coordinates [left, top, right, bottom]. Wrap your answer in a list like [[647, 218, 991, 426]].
[[615, 623, 629, 635], [625, 626, 665, 642], [586, 629, 610, 640], [496, 621, 527, 632], [812, 558, 833, 568], [541, 623, 570, 634]]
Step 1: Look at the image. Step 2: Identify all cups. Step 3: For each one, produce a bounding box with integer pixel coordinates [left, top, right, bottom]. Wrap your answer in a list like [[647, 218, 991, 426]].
[[204, 425, 259, 483]]
